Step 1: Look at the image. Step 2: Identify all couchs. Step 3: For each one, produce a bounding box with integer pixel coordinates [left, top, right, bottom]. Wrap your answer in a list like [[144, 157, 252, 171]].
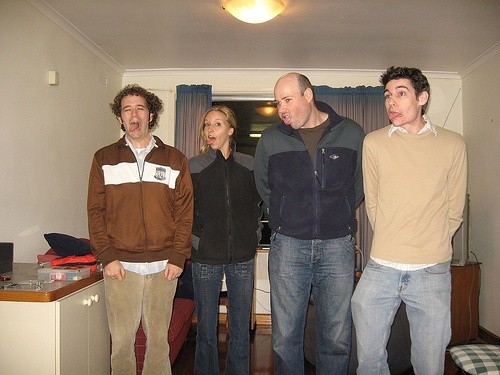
[[37, 238, 194, 375]]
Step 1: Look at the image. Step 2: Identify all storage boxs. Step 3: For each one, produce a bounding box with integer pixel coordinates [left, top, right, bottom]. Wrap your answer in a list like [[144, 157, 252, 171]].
[[38, 266, 90, 281]]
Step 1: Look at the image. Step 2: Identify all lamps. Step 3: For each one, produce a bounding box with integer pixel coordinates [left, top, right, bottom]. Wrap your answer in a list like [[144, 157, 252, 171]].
[[222, 0, 285, 24]]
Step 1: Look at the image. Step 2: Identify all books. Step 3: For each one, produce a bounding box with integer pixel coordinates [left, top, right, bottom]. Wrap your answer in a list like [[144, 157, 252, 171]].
[[37, 267, 90, 281]]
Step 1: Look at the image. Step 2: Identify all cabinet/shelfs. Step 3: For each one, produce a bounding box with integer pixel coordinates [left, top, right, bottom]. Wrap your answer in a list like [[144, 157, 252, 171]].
[[0, 262, 110, 375]]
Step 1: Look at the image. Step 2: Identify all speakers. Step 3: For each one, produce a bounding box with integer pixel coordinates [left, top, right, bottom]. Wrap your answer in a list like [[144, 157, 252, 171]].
[[451, 194, 470, 265]]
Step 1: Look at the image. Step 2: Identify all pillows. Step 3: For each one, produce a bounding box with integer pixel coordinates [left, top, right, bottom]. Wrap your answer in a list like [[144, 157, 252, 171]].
[[43, 233, 92, 258]]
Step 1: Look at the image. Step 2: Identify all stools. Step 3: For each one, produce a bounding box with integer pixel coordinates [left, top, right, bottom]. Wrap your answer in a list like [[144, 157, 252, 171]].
[[445, 343, 500, 375]]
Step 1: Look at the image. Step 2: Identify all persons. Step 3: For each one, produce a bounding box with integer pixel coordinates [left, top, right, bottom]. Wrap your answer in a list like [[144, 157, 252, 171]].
[[254, 72, 365, 375], [188, 107, 264, 375], [87, 84, 193, 375], [351, 66, 467, 375]]
